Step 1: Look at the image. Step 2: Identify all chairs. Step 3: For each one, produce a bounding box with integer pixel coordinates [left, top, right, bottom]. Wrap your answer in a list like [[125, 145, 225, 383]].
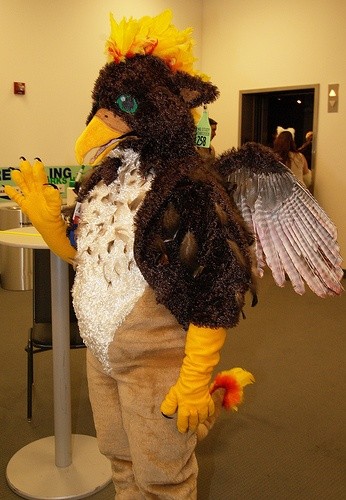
[[24, 227, 88, 423]]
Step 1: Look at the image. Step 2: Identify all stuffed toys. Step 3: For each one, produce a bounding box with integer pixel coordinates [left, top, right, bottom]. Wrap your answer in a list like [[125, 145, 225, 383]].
[[2, 8, 344, 500]]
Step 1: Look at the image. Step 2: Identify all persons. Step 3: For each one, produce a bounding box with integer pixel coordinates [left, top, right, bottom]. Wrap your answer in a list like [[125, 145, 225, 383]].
[[197, 119, 313, 189]]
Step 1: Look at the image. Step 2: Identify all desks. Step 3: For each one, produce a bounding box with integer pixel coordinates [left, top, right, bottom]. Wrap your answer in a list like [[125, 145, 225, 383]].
[[0, 226, 113, 500]]
